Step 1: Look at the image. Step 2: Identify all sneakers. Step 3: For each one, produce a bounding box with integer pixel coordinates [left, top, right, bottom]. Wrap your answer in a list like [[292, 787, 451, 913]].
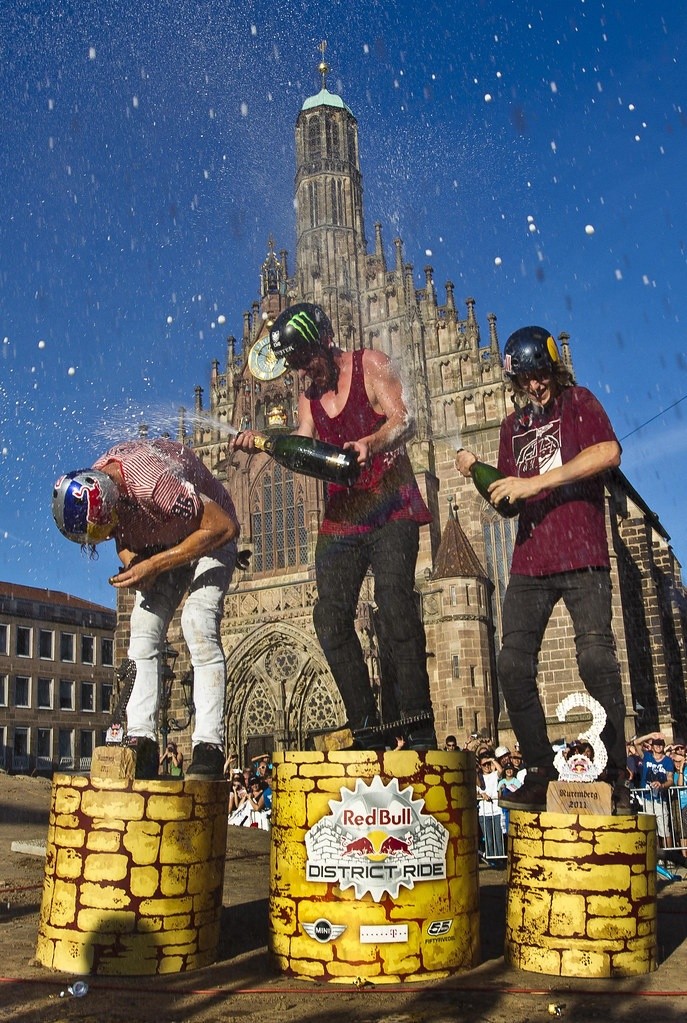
[[330, 718, 383, 751], [186, 743, 228, 779], [398, 711, 438, 751], [499, 768, 551, 809], [601, 768, 635, 814], [120, 737, 157, 778]]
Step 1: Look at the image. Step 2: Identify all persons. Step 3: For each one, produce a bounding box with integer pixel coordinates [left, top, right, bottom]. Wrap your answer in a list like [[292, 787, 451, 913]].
[[228, 303, 437, 751], [457, 324, 647, 815], [43, 434, 242, 791], [96, 718, 687, 869]]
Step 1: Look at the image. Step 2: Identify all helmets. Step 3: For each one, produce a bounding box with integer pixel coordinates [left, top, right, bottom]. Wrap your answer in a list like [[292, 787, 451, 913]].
[[52, 469, 122, 542], [270, 304, 337, 360], [502, 326, 561, 384]]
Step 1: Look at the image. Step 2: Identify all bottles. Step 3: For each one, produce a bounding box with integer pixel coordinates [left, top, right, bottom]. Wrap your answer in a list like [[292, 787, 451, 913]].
[[235, 429, 362, 487], [457, 449, 525, 519]]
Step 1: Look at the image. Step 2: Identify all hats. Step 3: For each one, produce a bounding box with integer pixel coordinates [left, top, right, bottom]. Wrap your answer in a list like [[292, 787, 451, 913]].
[[162, 736, 687, 810]]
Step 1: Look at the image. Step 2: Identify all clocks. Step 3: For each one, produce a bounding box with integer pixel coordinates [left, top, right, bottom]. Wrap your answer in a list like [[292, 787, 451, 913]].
[[248, 335, 288, 381]]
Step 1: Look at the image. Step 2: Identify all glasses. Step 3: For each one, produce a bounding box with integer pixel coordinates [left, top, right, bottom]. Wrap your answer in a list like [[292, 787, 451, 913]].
[[284, 343, 321, 373]]
